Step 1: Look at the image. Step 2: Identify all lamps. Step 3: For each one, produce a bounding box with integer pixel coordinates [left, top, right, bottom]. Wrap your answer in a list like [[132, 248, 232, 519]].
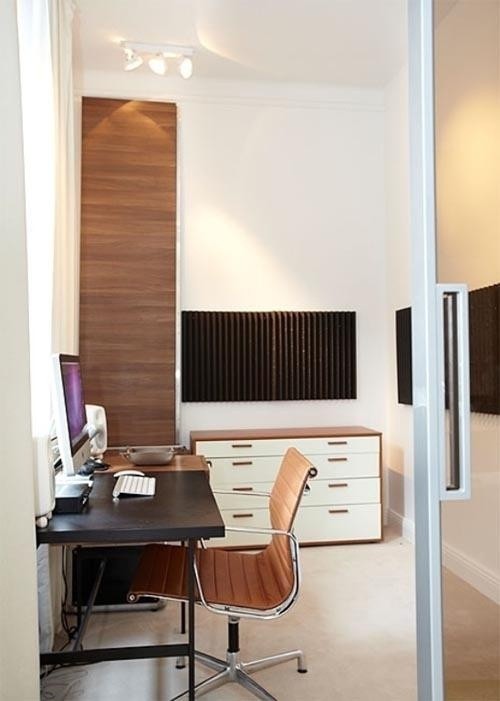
[[121, 40, 196, 78]]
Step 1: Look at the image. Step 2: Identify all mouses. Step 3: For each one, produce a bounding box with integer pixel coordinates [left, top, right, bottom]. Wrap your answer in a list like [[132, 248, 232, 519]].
[[113, 470, 146, 478]]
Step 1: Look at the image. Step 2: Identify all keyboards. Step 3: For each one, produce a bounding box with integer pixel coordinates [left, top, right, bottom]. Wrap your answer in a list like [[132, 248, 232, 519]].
[[111, 476, 156, 498]]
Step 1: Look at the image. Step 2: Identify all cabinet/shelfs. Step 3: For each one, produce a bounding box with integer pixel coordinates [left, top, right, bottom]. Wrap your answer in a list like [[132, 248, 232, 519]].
[[188, 424, 385, 554]]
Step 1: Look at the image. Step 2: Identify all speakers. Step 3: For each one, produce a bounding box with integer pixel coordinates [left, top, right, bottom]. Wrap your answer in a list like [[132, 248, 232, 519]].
[[33, 433, 57, 528], [84, 404, 108, 464]]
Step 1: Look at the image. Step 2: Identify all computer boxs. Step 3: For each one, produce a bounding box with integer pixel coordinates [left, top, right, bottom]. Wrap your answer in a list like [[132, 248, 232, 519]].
[[72, 544, 167, 612]]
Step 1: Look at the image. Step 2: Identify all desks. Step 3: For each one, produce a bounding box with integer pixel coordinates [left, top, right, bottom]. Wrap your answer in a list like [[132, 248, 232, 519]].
[[35, 471, 225, 699], [94, 455, 211, 471]]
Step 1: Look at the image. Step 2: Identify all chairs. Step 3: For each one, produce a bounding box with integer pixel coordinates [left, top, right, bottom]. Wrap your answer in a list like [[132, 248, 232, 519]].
[[125, 445, 319, 701]]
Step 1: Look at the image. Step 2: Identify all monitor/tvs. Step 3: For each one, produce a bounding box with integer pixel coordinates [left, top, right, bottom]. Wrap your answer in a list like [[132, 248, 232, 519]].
[[44, 352, 94, 488]]
